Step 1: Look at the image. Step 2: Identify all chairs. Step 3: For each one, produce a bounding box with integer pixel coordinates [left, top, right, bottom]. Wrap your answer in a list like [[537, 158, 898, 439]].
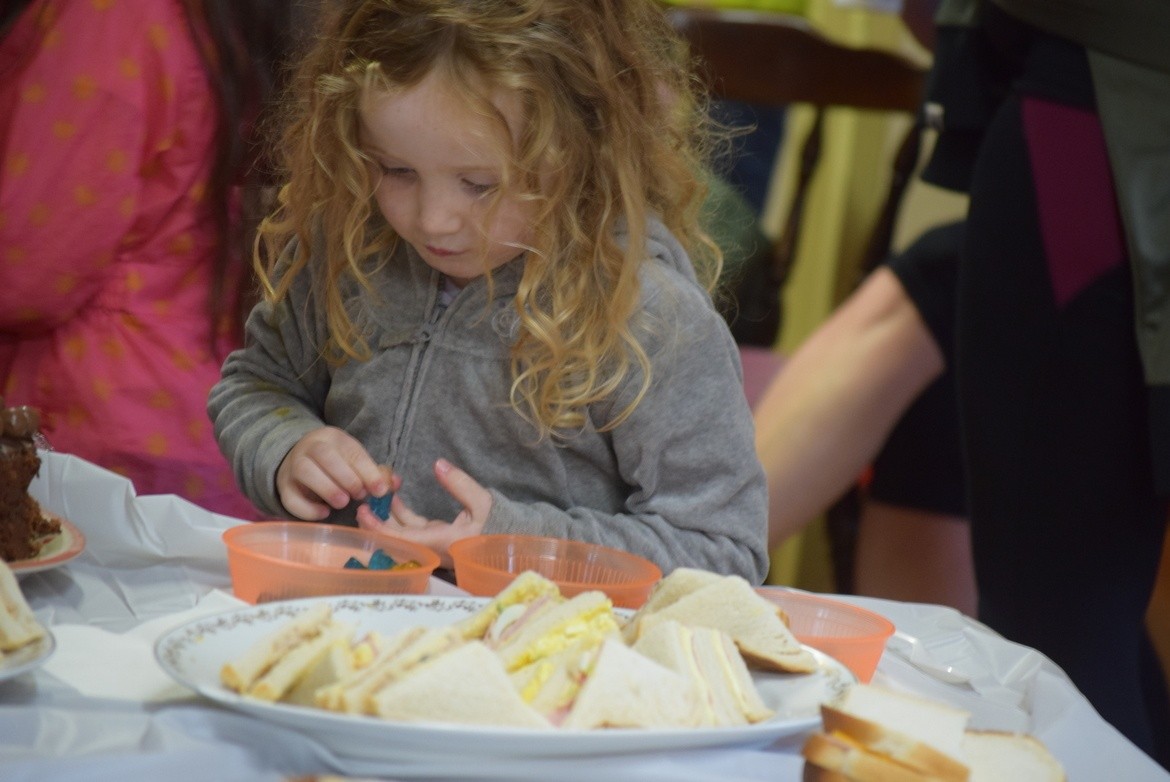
[[681, 14, 934, 588]]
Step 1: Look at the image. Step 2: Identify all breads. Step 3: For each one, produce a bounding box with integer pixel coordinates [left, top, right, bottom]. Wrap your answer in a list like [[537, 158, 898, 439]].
[[799, 679, 1065, 782]]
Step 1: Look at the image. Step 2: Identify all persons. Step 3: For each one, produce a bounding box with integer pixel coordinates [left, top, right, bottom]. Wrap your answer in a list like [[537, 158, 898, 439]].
[[718, 0, 1170, 771], [202, 0, 775, 587], [1, 1, 276, 524]]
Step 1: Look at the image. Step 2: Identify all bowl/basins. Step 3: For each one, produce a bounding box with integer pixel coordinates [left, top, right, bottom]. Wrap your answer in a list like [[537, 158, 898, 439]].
[[446, 534, 660, 611], [220, 521, 440, 605], [757, 589, 894, 685]]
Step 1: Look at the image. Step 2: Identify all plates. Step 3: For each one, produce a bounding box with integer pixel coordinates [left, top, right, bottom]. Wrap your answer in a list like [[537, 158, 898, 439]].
[[155, 592, 858, 768], [2, 622, 57, 678], [5, 510, 89, 582]]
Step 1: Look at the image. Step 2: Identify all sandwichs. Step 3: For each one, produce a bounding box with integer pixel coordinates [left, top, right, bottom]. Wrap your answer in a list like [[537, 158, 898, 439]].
[[223, 561, 816, 735]]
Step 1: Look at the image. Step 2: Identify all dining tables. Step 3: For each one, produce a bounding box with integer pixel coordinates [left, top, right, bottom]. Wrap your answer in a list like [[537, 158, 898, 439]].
[[0, 446, 1170, 782]]
